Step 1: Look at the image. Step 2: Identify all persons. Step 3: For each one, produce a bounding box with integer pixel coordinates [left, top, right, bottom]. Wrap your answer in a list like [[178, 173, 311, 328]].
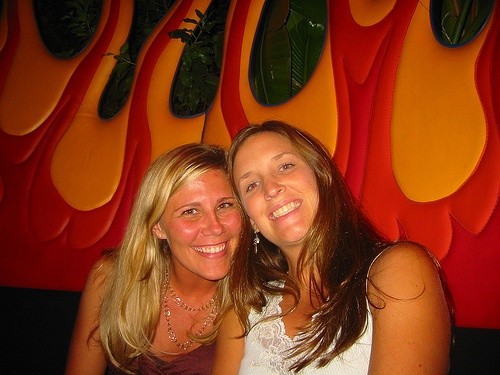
[[211, 120, 451, 375], [65, 143, 267, 375]]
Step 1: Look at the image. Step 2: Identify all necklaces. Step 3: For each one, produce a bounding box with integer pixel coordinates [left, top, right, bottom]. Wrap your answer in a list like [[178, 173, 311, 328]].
[[166, 274, 223, 310], [161, 303, 214, 350]]
